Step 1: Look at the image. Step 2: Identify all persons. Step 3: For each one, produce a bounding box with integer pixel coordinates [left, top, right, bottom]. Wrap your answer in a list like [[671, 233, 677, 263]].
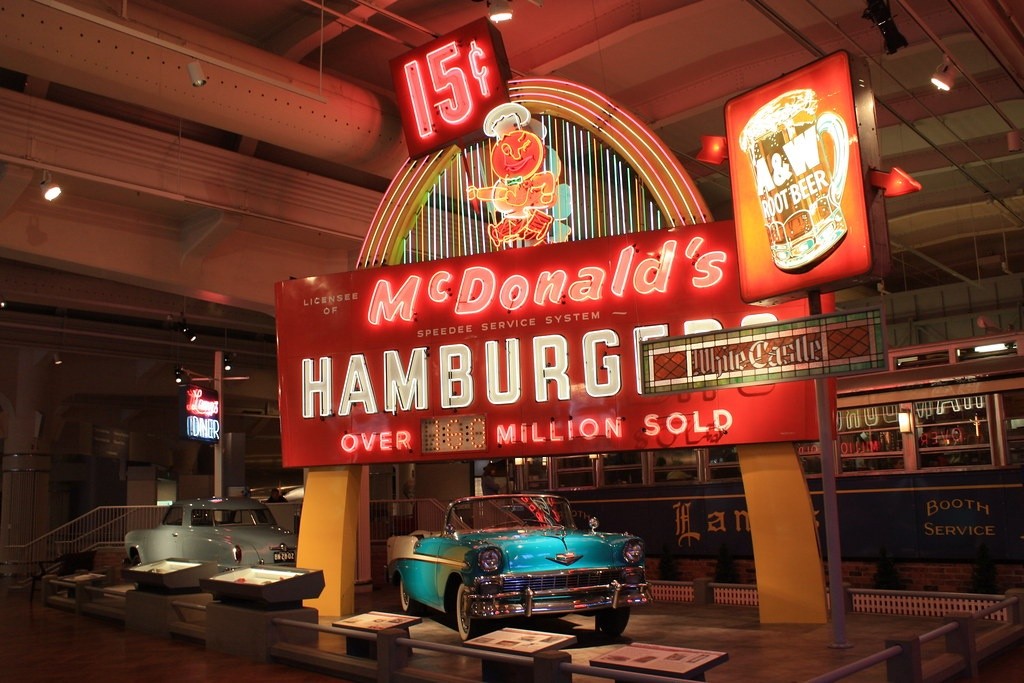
[[482, 465, 499, 496], [852, 431, 991, 469], [264, 488, 288, 503], [654, 457, 692, 480]]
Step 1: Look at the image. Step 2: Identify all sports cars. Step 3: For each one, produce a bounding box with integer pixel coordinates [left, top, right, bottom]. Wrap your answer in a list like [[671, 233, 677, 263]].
[[385, 493, 652, 646], [125, 496, 298, 572]]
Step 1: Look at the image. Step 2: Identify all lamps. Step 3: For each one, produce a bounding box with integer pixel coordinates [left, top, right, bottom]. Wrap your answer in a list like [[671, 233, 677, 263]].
[[174, 368, 183, 383], [931, 58, 957, 92], [898, 408, 913, 433], [1007, 130, 1022, 152], [40, 170, 61, 202], [487, 0, 513, 23], [225, 354, 233, 371], [188, 60, 207, 88], [177, 319, 197, 343]]
